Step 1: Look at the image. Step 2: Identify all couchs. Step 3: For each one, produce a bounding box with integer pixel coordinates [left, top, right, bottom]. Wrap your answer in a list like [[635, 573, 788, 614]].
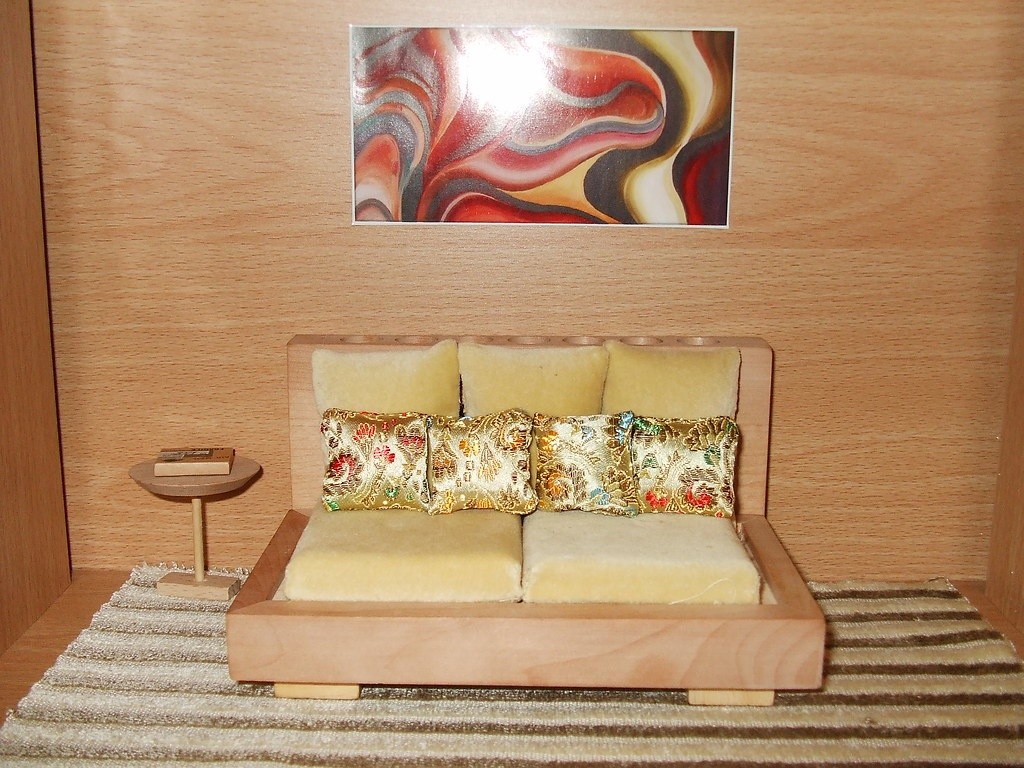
[[225, 336, 827, 708]]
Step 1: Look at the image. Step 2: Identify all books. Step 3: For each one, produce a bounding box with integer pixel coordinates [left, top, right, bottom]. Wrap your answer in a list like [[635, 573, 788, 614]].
[[154, 447, 235, 475]]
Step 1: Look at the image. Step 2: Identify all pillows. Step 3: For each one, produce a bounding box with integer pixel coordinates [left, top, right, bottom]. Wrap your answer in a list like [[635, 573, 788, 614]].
[[319, 408, 740, 518]]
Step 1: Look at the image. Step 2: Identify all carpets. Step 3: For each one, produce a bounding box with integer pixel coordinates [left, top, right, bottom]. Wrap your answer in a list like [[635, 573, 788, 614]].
[[0, 562, 1023, 767]]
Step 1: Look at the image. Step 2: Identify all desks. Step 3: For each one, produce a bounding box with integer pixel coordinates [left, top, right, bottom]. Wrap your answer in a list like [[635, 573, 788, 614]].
[[128, 454, 261, 602]]
